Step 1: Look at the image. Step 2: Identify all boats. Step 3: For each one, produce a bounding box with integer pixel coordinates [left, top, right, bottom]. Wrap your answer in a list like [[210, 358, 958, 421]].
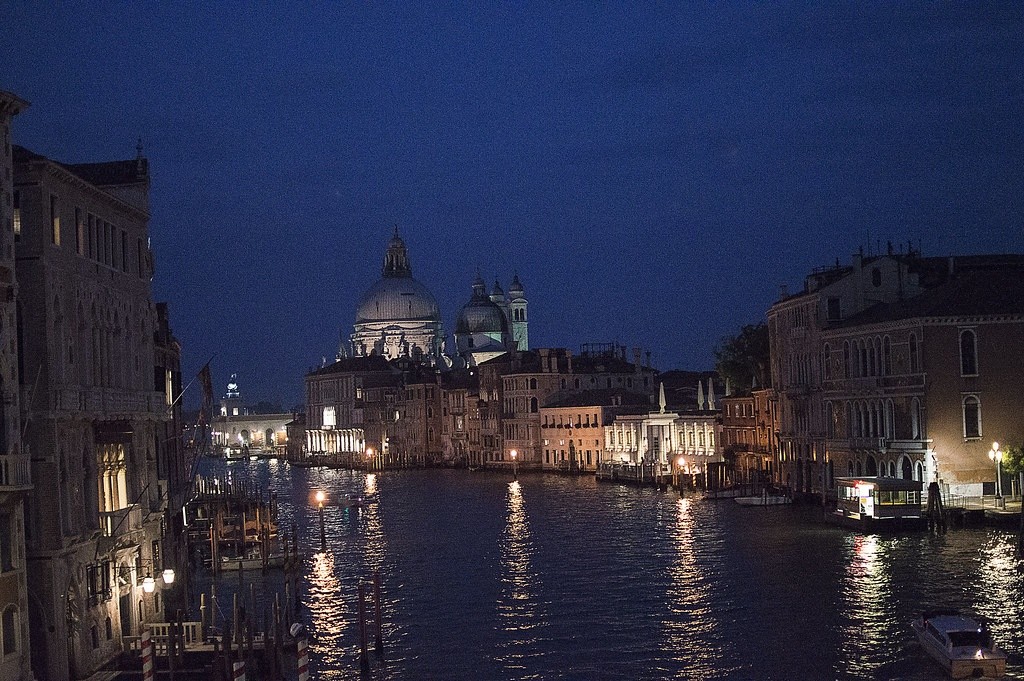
[[911, 605, 1009, 681]]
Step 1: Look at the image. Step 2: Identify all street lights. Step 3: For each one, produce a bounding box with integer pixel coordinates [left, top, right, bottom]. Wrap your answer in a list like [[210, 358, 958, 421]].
[[989, 442, 1003, 499]]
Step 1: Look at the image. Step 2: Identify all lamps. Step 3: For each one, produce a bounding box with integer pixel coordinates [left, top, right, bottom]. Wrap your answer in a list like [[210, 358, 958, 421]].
[[114, 558, 176, 584], [112, 565, 155, 593]]
